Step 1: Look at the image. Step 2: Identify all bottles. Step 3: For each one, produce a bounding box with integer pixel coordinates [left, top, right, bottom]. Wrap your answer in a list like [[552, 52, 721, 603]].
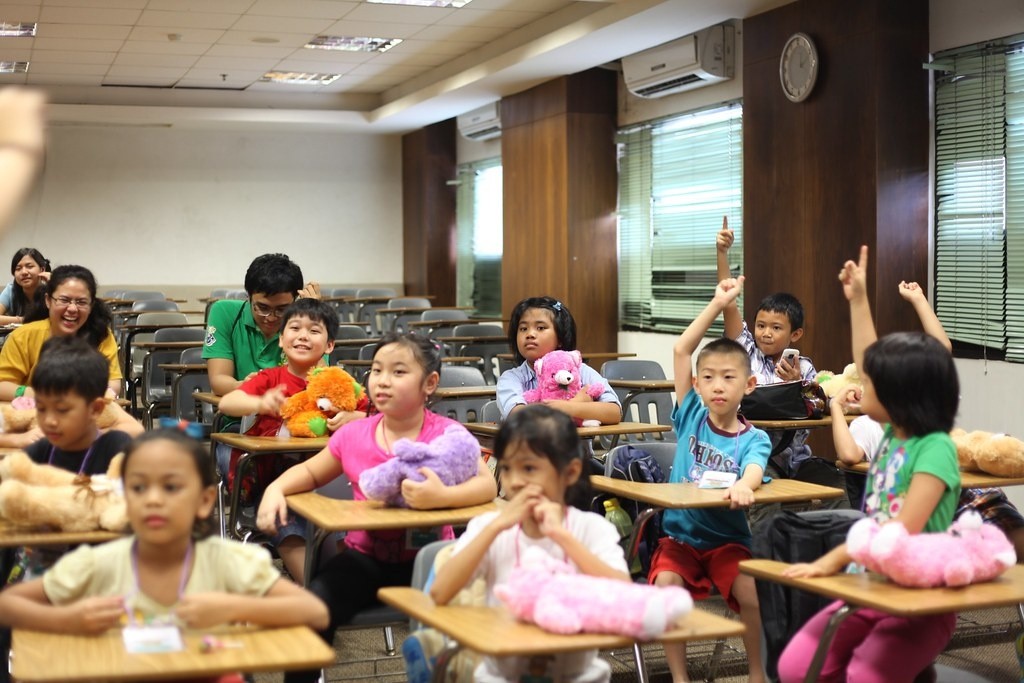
[[604, 499, 642, 575], [4, 549, 32, 587]]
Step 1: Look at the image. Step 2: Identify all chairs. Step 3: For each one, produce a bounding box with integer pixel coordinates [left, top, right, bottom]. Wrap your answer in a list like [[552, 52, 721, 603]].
[[0, 281, 1024, 683]]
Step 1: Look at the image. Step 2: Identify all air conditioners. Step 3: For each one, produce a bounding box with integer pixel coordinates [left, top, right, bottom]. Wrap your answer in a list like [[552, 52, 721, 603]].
[[621, 25, 736, 99], [456, 102, 503, 143]]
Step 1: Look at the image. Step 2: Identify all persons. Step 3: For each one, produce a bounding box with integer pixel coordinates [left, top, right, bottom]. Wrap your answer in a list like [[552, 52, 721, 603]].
[[0, 216, 962, 683]]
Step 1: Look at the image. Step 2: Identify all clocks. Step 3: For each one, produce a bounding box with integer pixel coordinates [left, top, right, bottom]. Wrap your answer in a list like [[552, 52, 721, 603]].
[[780, 31, 819, 103]]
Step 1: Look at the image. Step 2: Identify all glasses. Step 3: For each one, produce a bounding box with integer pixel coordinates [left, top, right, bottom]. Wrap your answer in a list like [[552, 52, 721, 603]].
[[51, 296, 90, 309], [252, 304, 289, 318]]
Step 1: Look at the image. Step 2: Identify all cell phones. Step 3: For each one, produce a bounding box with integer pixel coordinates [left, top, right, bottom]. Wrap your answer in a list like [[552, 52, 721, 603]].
[[778, 348, 799, 371]]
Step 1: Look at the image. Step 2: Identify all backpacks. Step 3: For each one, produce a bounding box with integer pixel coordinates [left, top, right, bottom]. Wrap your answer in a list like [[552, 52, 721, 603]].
[[568, 445, 666, 581], [749, 503, 863, 681], [740, 379, 828, 456], [951, 487, 1024, 563]]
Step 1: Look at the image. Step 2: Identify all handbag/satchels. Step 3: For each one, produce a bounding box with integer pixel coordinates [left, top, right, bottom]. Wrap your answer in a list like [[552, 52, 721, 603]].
[[781, 455, 869, 512]]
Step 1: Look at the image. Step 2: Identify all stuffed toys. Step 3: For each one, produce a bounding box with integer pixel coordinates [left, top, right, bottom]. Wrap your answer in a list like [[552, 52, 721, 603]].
[[847, 510, 1017, 588], [493, 552, 693, 642], [0, 452, 131, 533], [359, 424, 481, 508], [522, 350, 604, 425], [810, 363, 866, 416], [0, 395, 118, 429], [279, 365, 366, 439], [948, 427, 1024, 477]]
